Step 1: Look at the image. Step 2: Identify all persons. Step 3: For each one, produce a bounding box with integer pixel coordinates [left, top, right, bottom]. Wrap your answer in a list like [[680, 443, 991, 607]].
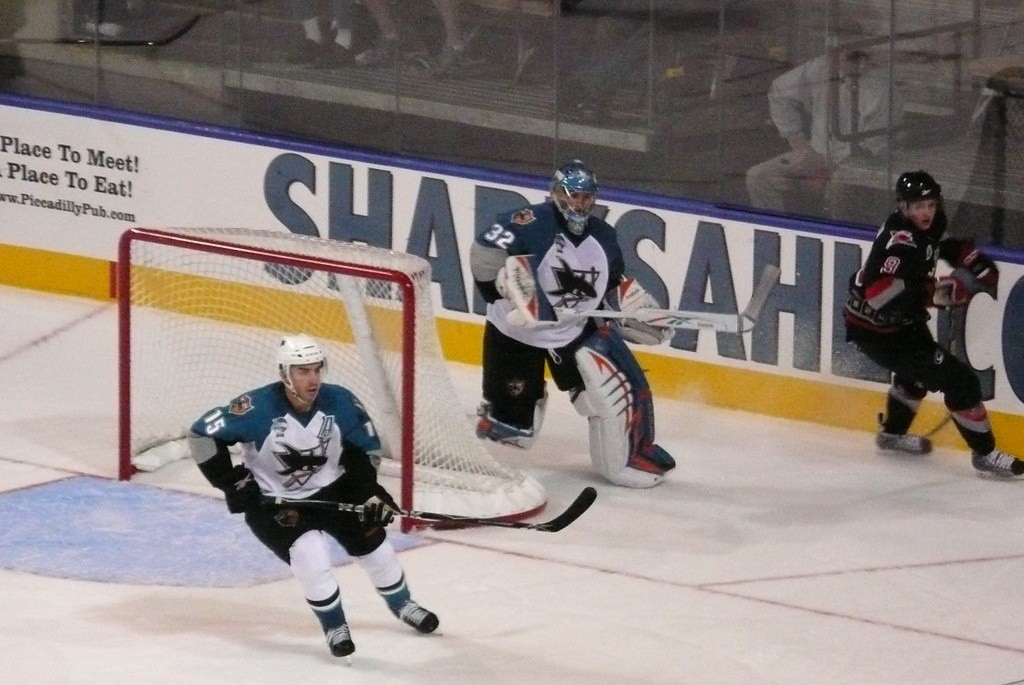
[[187, 334, 440, 657], [469, 159, 676, 489], [745, 15, 907, 223], [298, 1, 811, 202], [843, 171, 1024, 480]]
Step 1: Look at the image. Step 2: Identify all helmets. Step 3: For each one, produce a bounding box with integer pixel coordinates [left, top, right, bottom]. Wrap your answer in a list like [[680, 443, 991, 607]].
[[549, 160, 599, 235], [896, 170, 942, 202], [276, 333, 323, 375]]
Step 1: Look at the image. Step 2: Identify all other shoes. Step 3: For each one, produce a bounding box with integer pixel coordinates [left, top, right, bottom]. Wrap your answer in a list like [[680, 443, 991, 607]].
[[285, 37, 323, 64], [316, 41, 349, 66], [355, 40, 403, 66], [510, 46, 537, 87], [433, 27, 479, 75], [84, 18, 124, 35], [568, 90, 602, 124]]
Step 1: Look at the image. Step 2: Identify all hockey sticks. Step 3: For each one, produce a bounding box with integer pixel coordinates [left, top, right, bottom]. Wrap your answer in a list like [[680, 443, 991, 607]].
[[266, 480, 600, 534], [552, 263, 783, 336], [924, 307, 958, 437]]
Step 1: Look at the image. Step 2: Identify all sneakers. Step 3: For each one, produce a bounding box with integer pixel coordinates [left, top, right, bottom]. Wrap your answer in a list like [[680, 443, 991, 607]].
[[322, 617, 356, 666], [972, 446, 1024, 479], [389, 600, 443, 636], [875, 414, 932, 456]]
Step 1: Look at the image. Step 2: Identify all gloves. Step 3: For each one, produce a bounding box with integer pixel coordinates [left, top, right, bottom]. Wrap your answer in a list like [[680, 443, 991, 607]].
[[932, 276, 968, 306], [961, 243, 999, 288], [224, 466, 264, 513], [359, 485, 401, 526]]
